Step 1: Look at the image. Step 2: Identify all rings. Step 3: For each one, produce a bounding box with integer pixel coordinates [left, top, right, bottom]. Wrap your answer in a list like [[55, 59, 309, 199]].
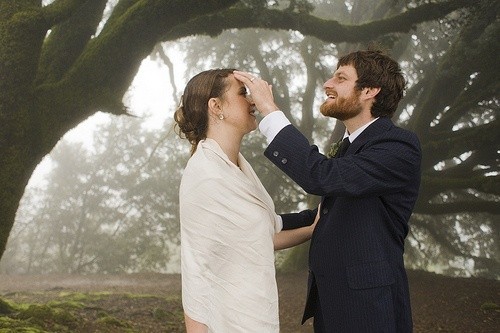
[[251, 78, 254, 82]]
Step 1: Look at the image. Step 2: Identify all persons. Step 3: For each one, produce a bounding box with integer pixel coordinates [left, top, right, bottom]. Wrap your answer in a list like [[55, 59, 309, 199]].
[[233, 50, 422, 333], [173, 68, 321, 332]]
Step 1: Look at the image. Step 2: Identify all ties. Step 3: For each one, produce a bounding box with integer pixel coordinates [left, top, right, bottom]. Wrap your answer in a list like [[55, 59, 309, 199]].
[[335, 137, 350, 158]]
